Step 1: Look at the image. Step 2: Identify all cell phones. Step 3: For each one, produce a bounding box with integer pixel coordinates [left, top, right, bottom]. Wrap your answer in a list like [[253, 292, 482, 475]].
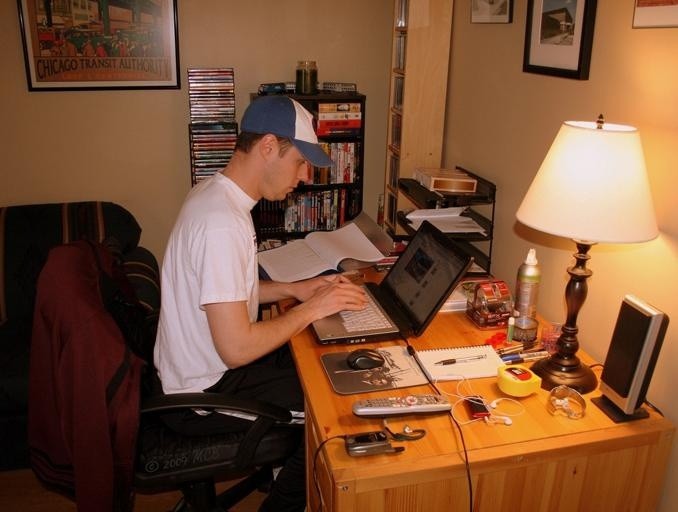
[[345, 430, 405, 455]]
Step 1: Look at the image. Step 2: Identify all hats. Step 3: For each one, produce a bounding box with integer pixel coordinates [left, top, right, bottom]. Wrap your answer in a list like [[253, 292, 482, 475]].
[[238, 95, 338, 169]]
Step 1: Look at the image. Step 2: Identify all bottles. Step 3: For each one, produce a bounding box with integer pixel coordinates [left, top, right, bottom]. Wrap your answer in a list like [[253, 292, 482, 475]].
[[515, 247, 542, 321], [506, 317, 515, 342], [295, 60, 319, 98]]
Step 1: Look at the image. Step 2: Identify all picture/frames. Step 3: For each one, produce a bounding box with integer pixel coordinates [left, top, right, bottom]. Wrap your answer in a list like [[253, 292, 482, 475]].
[[522, 0, 597, 80], [471, 0, 513, 24], [632, 0, 678, 29], [17, 0, 180, 92]]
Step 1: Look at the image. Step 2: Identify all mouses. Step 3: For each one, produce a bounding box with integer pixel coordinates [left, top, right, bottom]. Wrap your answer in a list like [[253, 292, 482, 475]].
[[348, 349, 385, 369]]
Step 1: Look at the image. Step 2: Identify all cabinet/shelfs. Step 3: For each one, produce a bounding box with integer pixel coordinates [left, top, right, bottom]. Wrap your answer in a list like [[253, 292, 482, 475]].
[[397, 165, 496, 281], [249, 92, 366, 241], [383, 0, 454, 243]]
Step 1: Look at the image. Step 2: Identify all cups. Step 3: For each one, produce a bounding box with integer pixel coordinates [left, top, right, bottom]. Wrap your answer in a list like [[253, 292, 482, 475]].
[[514, 316, 538, 343]]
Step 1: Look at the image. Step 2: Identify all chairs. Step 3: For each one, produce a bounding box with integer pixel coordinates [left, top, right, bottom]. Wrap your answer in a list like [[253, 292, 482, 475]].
[[1, 201, 160, 472], [41, 241, 298, 512]]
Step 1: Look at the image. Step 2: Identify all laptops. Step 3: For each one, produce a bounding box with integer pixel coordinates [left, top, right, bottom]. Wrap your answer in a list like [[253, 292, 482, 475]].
[[311, 220, 474, 344]]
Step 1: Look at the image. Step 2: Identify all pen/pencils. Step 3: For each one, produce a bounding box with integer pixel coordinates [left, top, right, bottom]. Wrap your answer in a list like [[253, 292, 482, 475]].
[[433, 354, 486, 366], [496, 341, 552, 365]]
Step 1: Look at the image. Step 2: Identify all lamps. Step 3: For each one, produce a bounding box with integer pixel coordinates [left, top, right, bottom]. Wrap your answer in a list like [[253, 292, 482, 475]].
[[514, 112, 658, 395]]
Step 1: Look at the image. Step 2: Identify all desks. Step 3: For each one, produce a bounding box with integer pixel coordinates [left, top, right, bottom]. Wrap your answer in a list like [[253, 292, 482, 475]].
[[260, 239, 678, 512]]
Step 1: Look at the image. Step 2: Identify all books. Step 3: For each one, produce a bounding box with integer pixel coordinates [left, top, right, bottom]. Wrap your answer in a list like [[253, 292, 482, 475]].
[[414, 345, 506, 382], [261, 103, 362, 233]]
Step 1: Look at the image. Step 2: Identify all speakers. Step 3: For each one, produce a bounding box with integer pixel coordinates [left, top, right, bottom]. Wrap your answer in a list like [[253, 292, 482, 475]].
[[590, 294, 670, 423]]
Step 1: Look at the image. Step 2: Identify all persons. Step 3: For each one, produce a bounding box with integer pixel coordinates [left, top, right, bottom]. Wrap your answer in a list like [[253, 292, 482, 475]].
[[153, 69, 368, 512]]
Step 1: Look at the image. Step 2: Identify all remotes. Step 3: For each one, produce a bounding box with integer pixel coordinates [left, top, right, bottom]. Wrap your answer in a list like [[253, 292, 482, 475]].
[[351, 394, 451, 418]]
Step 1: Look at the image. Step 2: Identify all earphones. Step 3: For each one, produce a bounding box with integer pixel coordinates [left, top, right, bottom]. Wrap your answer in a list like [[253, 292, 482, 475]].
[[489, 398, 501, 409], [498, 416, 512, 425]]
